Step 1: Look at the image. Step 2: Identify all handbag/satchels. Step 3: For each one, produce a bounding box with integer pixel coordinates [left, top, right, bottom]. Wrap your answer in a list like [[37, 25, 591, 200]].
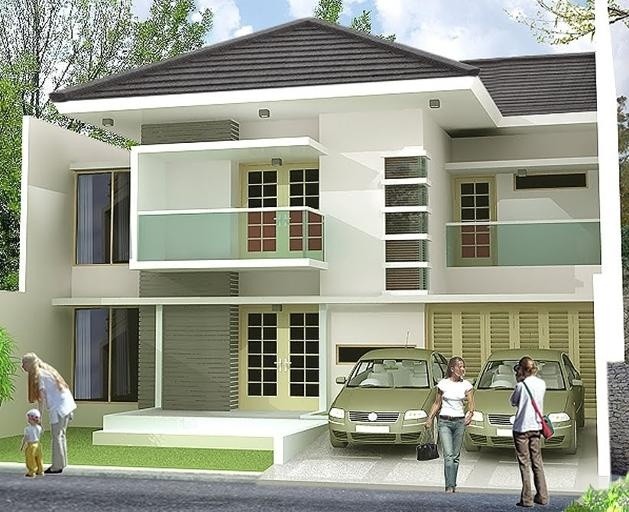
[[539, 412, 556, 441], [416, 428, 440, 460]]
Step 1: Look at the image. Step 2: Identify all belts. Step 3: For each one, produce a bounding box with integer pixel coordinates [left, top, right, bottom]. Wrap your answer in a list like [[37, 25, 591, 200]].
[[439, 414, 466, 422]]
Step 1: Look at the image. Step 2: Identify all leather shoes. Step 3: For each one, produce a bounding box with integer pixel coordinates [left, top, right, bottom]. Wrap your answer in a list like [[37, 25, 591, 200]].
[[25, 465, 63, 476]]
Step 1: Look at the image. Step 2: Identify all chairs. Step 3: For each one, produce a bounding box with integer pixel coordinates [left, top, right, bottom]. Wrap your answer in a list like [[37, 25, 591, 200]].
[[366, 359, 429, 386], [492, 364, 518, 388], [540, 364, 562, 389]]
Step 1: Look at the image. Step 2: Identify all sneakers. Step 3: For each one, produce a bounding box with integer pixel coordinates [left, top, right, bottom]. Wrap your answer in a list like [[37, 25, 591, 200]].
[[514, 497, 548, 507], [445, 487, 460, 493]]
